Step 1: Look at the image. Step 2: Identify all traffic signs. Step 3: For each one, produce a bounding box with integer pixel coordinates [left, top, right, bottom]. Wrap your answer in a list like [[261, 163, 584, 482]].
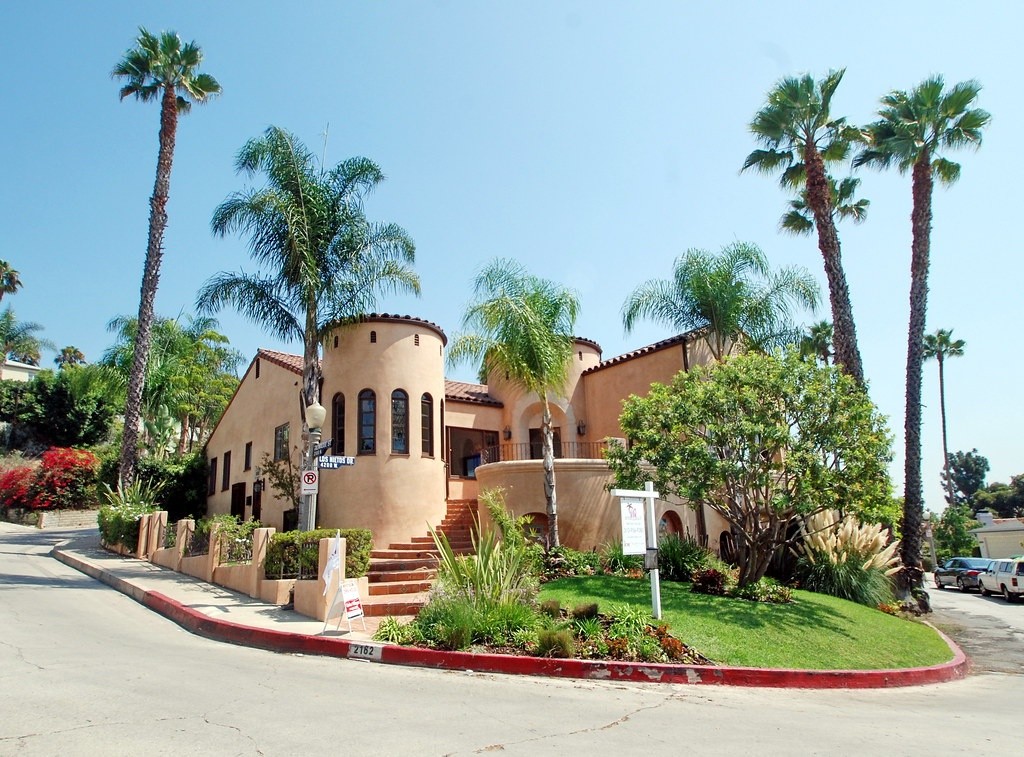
[[313, 437, 356, 469]]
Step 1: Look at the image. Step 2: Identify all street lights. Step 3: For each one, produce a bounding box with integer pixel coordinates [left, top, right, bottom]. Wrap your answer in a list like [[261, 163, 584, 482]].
[[925, 527, 939, 572], [301, 400, 327, 532]]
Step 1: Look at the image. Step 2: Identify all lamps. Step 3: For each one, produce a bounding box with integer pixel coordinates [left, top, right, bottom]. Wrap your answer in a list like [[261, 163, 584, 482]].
[[503, 425, 511, 441], [577, 419, 586, 436]]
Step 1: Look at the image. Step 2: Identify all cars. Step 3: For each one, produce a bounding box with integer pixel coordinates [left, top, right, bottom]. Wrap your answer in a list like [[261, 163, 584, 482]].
[[933, 557, 994, 592]]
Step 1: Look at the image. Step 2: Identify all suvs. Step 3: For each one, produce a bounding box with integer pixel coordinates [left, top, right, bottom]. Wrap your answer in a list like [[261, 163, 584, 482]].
[[976, 556, 1024, 603]]
